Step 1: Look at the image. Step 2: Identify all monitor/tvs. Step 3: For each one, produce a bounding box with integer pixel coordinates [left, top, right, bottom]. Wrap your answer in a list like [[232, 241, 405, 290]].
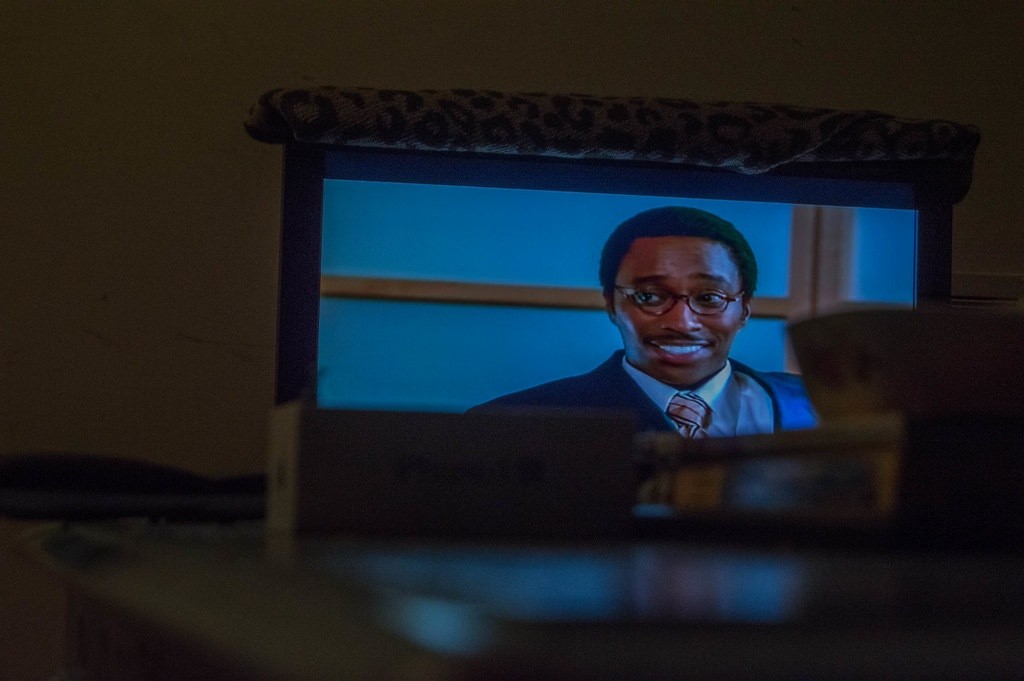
[[277, 142, 951, 437]]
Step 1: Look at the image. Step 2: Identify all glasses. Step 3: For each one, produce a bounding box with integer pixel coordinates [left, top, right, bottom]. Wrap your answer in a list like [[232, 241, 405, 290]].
[[613, 285, 745, 317]]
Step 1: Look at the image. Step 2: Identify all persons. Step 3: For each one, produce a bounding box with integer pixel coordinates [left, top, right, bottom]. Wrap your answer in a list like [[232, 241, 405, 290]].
[[461, 206, 821, 440]]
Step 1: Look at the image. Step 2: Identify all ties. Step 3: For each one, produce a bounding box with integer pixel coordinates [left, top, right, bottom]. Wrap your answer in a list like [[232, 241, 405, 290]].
[[666, 392, 715, 441]]
